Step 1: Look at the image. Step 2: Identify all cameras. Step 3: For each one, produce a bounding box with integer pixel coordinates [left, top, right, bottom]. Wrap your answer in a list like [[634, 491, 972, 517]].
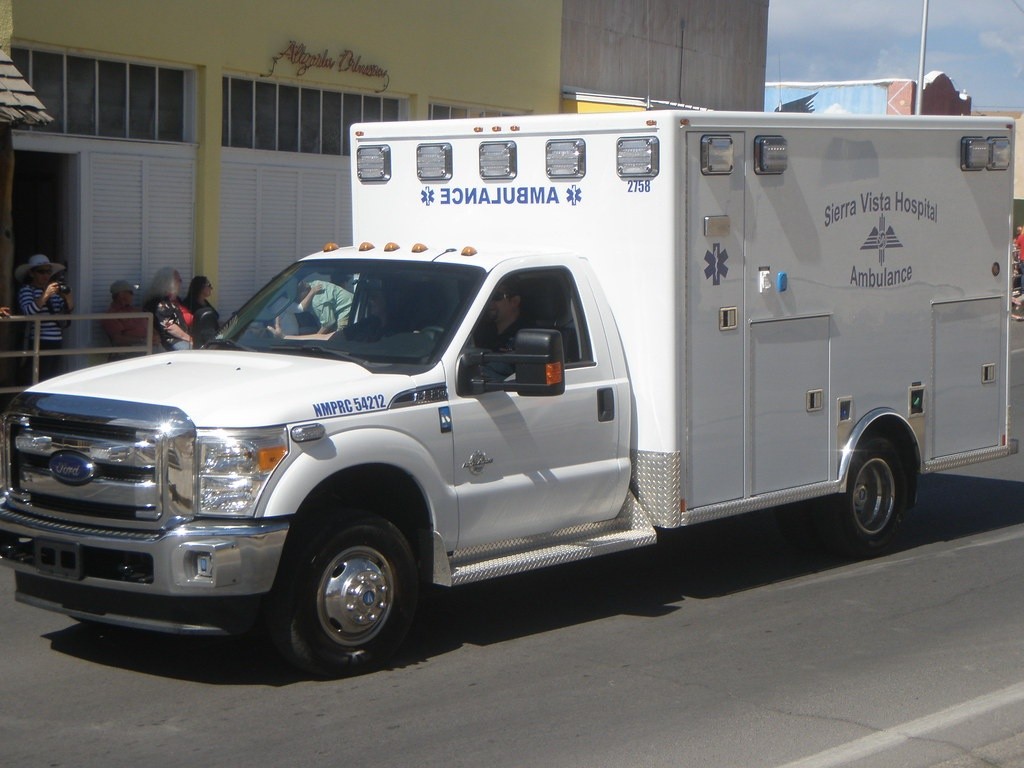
[[55, 281, 72, 295]]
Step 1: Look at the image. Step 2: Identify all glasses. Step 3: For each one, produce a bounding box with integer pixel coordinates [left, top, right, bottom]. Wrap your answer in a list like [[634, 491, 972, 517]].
[[204, 283, 212, 287], [492, 290, 518, 301], [33, 270, 53, 275], [174, 277, 182, 282], [126, 290, 134, 295]]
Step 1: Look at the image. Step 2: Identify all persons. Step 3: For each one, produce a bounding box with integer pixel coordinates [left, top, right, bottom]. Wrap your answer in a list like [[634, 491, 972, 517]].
[[146, 267, 196, 351], [187, 275, 226, 329], [15, 253, 75, 390], [0, 304, 11, 319], [267, 270, 431, 347], [283, 275, 324, 335], [104, 278, 169, 358], [283, 278, 367, 335], [415, 271, 562, 382]]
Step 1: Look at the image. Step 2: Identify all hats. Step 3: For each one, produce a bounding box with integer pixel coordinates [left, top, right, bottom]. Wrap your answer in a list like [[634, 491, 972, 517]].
[[15, 254, 65, 281], [110, 280, 141, 294]]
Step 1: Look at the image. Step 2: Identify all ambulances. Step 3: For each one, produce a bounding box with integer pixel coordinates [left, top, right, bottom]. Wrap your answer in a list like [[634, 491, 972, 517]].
[[0, 107, 1016, 678]]
